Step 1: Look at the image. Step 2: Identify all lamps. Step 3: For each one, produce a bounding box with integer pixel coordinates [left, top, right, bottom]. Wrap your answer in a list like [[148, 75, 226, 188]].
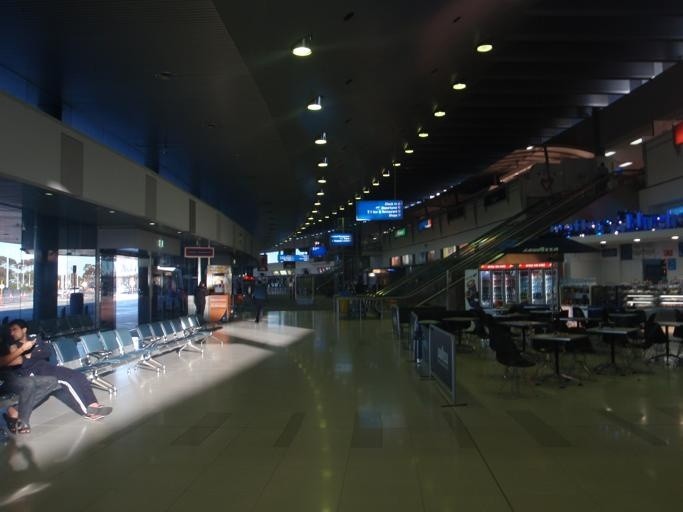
[[318, 176, 327, 183], [275, 226, 307, 247], [314, 132, 327, 145], [472, 31, 493, 53], [404, 143, 413, 154], [372, 178, 379, 186], [318, 156, 328, 167], [433, 105, 445, 117], [418, 127, 428, 138], [307, 96, 322, 111], [291, 32, 312, 57], [452, 72, 467, 90], [382, 168, 390, 177], [301, 187, 371, 227], [392, 159, 401, 167]]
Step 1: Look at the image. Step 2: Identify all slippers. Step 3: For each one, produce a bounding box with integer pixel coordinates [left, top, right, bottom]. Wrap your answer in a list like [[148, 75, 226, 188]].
[[2, 410, 31, 435]]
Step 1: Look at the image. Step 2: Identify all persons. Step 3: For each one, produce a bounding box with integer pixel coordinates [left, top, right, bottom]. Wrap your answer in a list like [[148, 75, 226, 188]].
[[194, 280, 209, 322], [253, 280, 267, 323], [0, 318, 114, 434]]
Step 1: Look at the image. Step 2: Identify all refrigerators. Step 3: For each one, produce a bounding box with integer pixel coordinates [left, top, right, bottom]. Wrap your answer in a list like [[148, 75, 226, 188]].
[[464, 261, 559, 310]]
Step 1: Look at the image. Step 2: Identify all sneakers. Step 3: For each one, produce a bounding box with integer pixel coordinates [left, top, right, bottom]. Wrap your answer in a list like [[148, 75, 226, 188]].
[[84, 403, 114, 421]]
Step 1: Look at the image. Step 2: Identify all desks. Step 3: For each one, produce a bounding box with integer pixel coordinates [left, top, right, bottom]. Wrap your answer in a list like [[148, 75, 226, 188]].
[[419, 320, 440, 326], [442, 316, 479, 354]]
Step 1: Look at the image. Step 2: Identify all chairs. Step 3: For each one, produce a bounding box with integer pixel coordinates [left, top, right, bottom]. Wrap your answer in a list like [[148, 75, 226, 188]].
[[0, 315, 223, 436], [469, 303, 683, 401]]
